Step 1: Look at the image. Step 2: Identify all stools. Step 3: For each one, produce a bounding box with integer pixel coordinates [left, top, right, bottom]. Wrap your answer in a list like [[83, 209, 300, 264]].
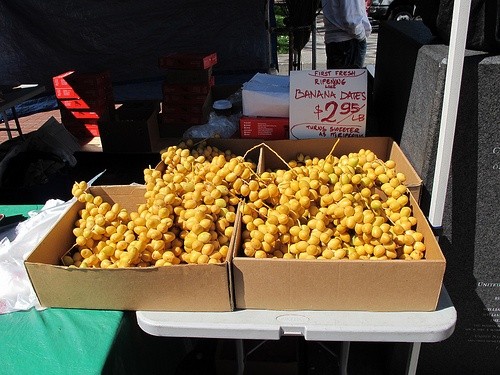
[[287, 24, 315, 70]]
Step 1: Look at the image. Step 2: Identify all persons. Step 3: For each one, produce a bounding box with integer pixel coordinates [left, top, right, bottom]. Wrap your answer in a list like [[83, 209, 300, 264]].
[[322, 0, 372, 70]]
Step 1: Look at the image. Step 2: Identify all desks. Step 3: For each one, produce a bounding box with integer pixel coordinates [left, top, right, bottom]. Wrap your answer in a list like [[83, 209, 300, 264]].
[[0, 204, 125, 375], [136, 280, 458, 375]]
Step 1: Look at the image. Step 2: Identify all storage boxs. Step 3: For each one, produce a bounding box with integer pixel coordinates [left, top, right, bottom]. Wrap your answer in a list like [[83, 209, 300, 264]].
[[23, 49, 447, 313]]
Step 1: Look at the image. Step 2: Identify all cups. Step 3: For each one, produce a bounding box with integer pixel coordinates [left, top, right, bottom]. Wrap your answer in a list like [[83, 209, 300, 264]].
[[213, 100, 232, 117]]
